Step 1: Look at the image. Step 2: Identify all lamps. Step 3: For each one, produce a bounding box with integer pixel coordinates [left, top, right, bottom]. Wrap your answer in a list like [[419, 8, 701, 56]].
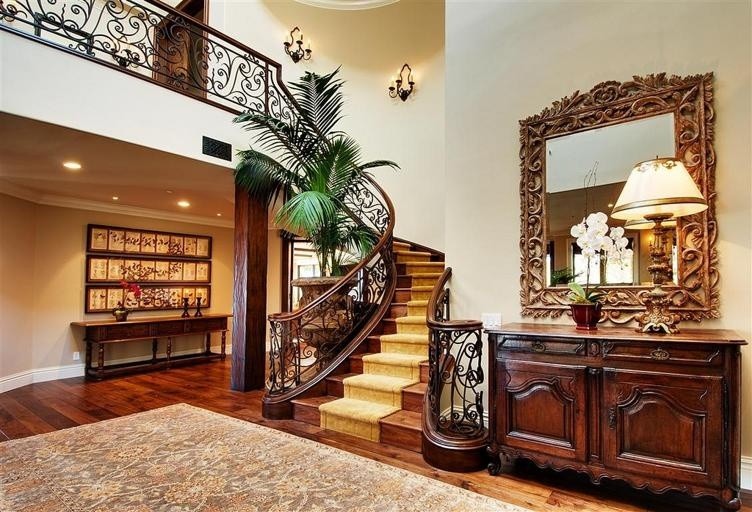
[[611, 154, 709, 335], [283, 24, 312, 64], [388, 62, 416, 101], [624, 219, 677, 286]]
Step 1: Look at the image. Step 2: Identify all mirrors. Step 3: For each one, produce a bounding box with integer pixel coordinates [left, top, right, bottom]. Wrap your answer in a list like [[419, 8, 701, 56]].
[[518, 69, 721, 323]]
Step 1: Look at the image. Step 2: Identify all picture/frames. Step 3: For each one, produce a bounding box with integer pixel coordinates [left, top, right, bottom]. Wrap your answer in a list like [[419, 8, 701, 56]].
[[85, 223, 212, 312]]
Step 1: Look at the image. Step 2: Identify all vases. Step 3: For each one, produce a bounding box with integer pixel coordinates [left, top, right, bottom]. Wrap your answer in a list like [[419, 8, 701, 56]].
[[568, 302, 603, 330], [113, 308, 128, 322]]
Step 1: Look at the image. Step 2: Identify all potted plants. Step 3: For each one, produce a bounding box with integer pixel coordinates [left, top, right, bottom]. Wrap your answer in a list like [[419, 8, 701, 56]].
[[231, 62, 402, 387]]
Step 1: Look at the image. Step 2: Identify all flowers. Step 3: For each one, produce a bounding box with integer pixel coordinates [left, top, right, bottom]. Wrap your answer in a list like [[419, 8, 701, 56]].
[[594, 226, 633, 285], [566, 211, 613, 303]]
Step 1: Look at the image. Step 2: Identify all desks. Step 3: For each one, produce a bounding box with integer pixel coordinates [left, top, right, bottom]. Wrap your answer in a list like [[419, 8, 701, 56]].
[[70, 314, 233, 381]]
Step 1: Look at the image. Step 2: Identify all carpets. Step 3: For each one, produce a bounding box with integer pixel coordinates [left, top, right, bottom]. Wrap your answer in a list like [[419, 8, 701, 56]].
[[0, 403, 528, 511]]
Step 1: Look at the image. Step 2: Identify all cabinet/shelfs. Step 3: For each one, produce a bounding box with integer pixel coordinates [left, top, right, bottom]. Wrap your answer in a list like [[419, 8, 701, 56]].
[[484, 322, 749, 512]]
